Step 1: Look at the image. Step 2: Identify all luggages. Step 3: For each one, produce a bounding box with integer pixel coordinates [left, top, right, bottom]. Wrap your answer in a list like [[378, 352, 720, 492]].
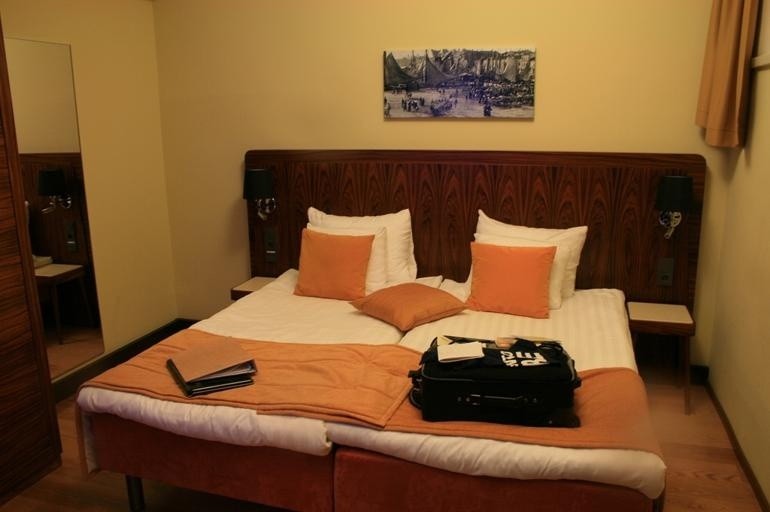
[[408, 335, 583, 430]]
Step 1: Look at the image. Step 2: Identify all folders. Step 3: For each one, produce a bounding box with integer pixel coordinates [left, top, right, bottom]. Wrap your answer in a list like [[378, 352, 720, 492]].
[[167, 338, 257, 397]]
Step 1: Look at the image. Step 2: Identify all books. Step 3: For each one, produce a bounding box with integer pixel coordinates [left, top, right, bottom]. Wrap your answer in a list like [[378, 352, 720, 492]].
[[165, 335, 260, 399]]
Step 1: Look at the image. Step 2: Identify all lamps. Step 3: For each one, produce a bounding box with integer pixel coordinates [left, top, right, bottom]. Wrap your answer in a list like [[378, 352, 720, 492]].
[[656, 173, 696, 242], [242, 165, 280, 233], [24, 157, 92, 216]]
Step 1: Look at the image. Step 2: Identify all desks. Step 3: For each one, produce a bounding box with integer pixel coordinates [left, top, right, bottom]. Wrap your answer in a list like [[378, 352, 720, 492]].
[[626, 300, 696, 418], [29, 262, 93, 348], [229, 277, 278, 300]]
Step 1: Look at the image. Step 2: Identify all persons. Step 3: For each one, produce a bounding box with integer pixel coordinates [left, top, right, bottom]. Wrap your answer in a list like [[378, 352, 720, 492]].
[[384, 77, 535, 118]]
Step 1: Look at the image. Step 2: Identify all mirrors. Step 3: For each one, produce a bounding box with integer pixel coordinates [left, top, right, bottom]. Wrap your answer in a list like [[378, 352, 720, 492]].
[[3, 31, 105, 384]]
